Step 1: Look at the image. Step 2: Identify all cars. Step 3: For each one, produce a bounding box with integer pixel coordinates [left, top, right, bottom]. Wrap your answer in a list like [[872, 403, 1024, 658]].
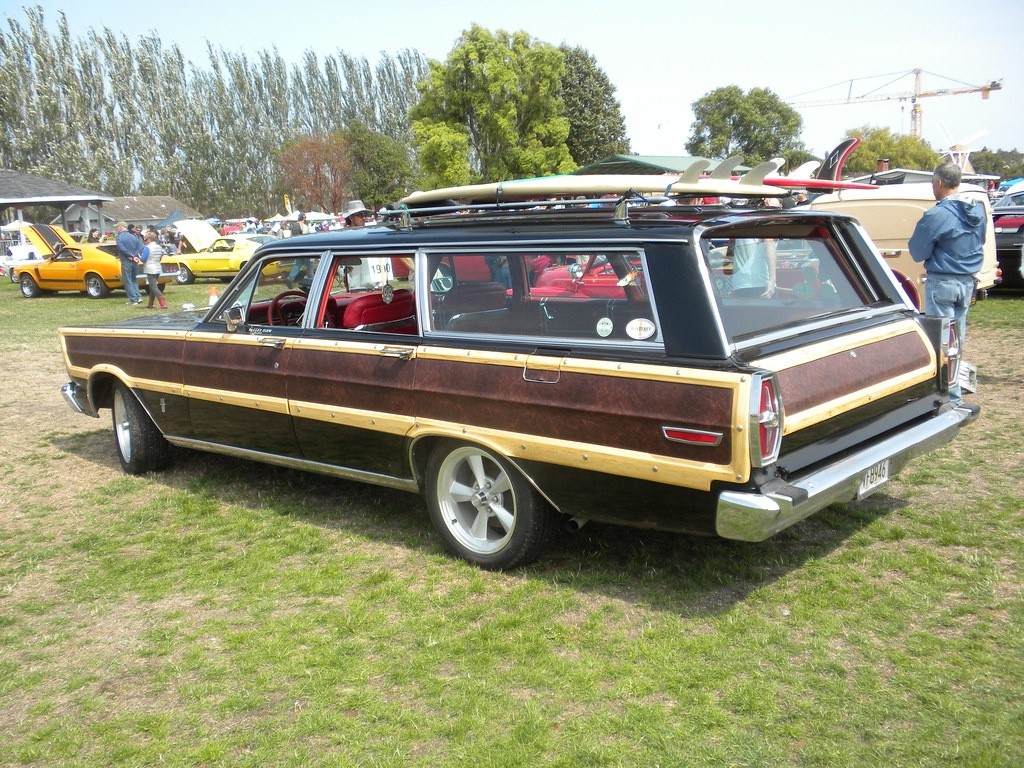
[[709, 181, 1004, 321], [14, 222, 182, 301], [986, 183, 1024, 291], [175, 233, 320, 287], [509, 228, 834, 307]]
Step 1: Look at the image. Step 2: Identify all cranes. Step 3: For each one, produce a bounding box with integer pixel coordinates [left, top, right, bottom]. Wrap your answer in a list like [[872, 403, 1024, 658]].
[[837, 68, 1003, 141]]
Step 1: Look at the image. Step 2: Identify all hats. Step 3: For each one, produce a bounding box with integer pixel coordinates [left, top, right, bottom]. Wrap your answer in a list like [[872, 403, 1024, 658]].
[[341, 200, 374, 218], [113, 221, 127, 227]]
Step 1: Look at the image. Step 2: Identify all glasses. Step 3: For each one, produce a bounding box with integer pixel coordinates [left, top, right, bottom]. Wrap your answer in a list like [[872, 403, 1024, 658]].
[[353, 213, 366, 219], [302, 217, 306, 219]]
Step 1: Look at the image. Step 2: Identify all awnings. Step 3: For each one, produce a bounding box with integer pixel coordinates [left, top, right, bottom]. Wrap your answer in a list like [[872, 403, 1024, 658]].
[[156, 209, 187, 230]]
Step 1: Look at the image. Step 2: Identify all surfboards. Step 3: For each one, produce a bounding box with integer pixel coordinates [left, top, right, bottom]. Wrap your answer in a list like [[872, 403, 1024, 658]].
[[397, 136, 879, 204]]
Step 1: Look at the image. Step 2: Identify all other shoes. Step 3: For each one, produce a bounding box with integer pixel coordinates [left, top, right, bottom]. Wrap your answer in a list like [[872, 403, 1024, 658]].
[[125, 302, 138, 305], [137, 297, 143, 303], [285, 278, 293, 289]]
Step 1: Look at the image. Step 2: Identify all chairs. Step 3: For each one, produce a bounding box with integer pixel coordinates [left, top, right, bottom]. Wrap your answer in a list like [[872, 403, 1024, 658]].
[[343, 289, 419, 336], [435, 280, 510, 333]]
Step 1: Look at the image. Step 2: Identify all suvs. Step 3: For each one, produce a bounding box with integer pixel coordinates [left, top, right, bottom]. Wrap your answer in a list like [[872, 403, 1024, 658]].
[[58, 170, 978, 575]]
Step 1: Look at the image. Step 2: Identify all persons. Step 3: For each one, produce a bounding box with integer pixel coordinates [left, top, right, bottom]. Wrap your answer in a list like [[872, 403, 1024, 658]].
[[146, 215, 377, 255], [908, 162, 987, 405], [113, 222, 144, 305], [87, 229, 107, 243], [422, 189, 808, 302], [988, 180, 995, 189], [138, 232, 167, 309], [287, 212, 315, 289], [336, 200, 382, 287]]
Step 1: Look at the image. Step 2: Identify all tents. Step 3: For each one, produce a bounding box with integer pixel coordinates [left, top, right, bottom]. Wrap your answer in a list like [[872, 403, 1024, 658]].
[[570, 153, 755, 175], [265, 210, 335, 223]]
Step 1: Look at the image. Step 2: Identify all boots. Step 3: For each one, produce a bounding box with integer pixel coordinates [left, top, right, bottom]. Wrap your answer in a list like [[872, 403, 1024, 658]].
[[157, 296, 167, 309], [146, 297, 155, 308]]
